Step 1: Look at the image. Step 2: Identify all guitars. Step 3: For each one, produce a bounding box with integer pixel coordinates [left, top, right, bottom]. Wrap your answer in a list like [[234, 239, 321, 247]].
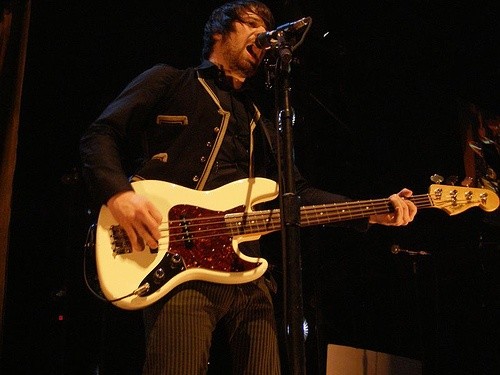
[[82, 173, 499, 310]]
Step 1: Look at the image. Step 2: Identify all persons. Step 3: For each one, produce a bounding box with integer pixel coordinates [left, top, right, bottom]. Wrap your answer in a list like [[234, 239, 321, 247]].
[[79, 0, 418, 375]]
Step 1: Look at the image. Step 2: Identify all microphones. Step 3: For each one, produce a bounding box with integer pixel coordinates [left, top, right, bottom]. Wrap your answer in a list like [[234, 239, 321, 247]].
[[391, 245, 433, 257], [257, 18, 307, 49]]
